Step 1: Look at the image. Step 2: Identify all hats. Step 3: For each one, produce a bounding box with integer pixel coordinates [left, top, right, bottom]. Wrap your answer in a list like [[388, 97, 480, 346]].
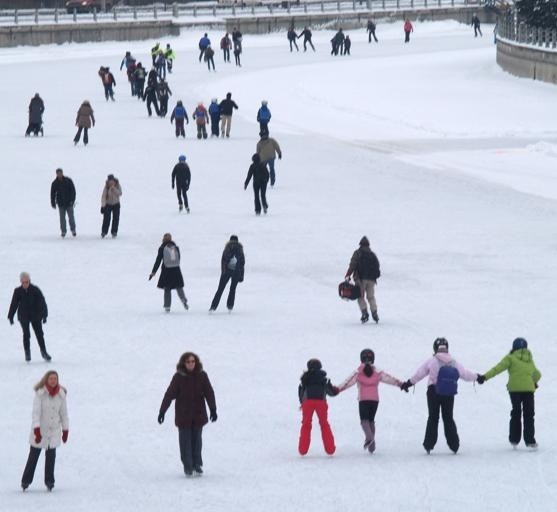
[[360, 349, 375, 363], [307, 359, 322, 369], [358, 235, 370, 246], [513, 337, 527, 350], [433, 337, 449, 354]]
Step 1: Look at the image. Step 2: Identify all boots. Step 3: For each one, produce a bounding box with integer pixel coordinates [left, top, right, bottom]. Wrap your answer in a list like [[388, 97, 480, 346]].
[[361, 309, 369, 321], [371, 310, 379, 321]]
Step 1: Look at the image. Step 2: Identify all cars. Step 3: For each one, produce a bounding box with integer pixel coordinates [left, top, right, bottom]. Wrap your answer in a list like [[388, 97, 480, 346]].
[[217, 0, 264, 7], [64, 0, 113, 14]]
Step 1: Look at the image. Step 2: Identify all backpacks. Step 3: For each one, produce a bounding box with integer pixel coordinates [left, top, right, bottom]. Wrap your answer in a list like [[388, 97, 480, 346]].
[[228, 245, 240, 271], [357, 250, 379, 280], [435, 356, 459, 396], [162, 242, 179, 269]]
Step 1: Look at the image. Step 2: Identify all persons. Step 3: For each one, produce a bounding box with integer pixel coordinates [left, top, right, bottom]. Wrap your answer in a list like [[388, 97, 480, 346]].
[[158, 352, 217, 475], [344, 236, 380, 322], [149, 232, 189, 310], [366, 20, 378, 43], [336, 349, 409, 452], [404, 18, 413, 42], [481, 338, 542, 447], [7, 272, 52, 361], [297, 359, 339, 455], [21, 370, 69, 489], [406, 337, 481, 452], [471, 12, 482, 37], [209, 235, 245, 312]]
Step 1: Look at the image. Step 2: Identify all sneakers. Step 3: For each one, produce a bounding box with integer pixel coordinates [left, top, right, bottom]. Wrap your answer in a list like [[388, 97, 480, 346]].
[[511, 440, 520, 445], [47, 482, 54, 489], [451, 446, 459, 452], [21, 481, 29, 489], [184, 469, 193, 476], [25, 353, 31, 361], [524, 441, 538, 448], [369, 448, 376, 453], [42, 351, 52, 360], [364, 438, 372, 448], [26, 131, 274, 239], [424, 446, 433, 451], [165, 305, 235, 311]]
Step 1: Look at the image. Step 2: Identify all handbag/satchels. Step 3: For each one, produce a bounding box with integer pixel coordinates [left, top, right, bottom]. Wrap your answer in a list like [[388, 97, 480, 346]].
[[338, 275, 361, 300]]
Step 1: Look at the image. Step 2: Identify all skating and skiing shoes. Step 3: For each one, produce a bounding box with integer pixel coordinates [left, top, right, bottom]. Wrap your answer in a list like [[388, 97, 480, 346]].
[[194, 467, 203, 473]]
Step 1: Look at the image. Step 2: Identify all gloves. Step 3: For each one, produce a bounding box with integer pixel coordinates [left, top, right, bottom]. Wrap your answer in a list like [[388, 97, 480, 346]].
[[149, 275, 152, 281], [158, 413, 164, 424], [477, 374, 485, 385], [345, 272, 351, 277], [401, 379, 412, 393], [210, 413, 218, 422], [10, 316, 14, 324], [43, 317, 47, 324], [62, 430, 69, 442], [34, 428, 42, 443]]
